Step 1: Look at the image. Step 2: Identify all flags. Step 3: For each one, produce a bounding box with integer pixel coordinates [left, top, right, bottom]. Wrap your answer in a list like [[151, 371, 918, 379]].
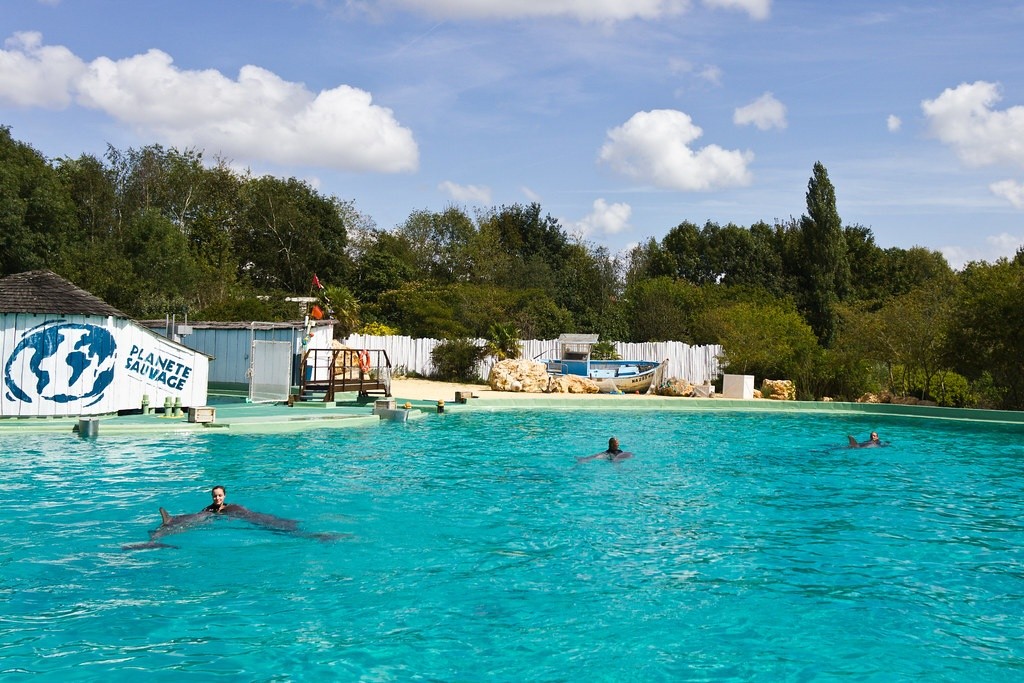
[[311, 306, 322, 319], [312, 273, 324, 289]]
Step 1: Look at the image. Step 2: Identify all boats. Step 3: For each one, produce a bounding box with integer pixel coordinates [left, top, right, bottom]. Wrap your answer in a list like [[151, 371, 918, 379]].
[[541, 358, 669, 394]]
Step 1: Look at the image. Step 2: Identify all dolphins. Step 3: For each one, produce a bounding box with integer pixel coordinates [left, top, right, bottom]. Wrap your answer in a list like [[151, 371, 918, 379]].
[[120, 503, 356, 549], [847, 434, 877, 449], [574, 451, 635, 463]]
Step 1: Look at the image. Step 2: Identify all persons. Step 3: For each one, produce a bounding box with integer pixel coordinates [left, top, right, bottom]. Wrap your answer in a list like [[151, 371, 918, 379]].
[[870, 432, 878, 447], [605, 438, 623, 457], [202, 486, 228, 514]]
